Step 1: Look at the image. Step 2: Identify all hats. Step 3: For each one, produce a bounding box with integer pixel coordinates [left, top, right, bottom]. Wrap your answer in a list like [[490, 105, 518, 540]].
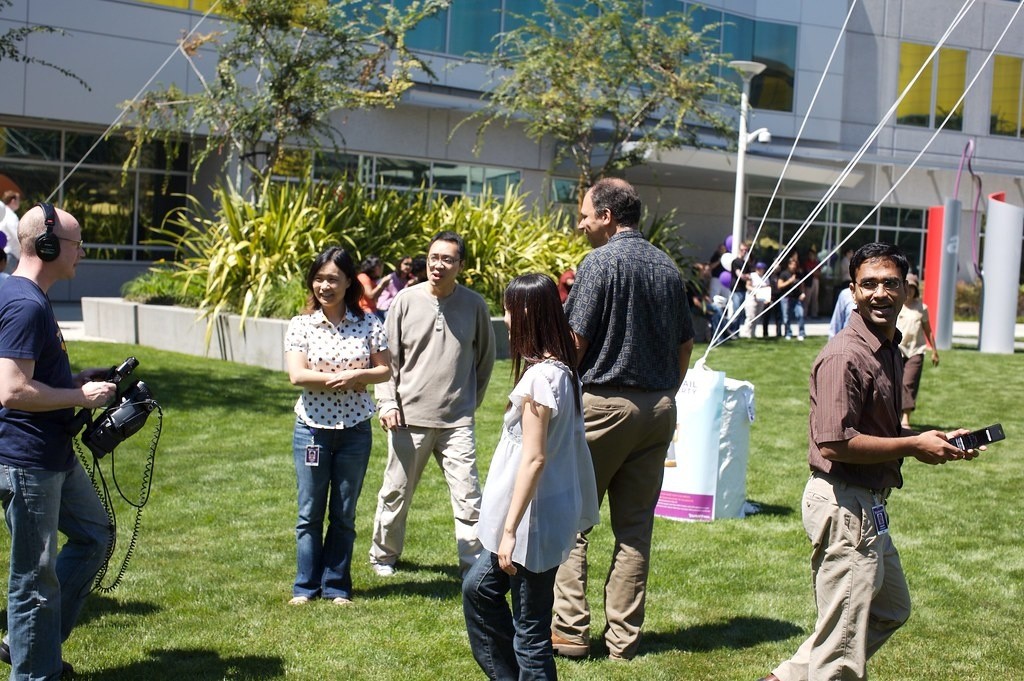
[[906, 273, 920, 286], [756, 262, 767, 270]]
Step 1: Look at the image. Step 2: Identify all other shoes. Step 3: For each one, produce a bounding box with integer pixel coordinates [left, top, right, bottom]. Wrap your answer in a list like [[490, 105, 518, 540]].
[[374, 565, 395, 578], [0, 642, 73, 681], [332, 597, 351, 607], [758, 674, 779, 681], [786, 336, 791, 340], [287, 596, 308, 606], [798, 336, 804, 340], [551, 633, 588, 655], [608, 652, 631, 662]]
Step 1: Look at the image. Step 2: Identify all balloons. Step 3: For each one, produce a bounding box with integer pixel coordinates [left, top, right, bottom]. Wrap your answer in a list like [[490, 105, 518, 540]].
[[0, 231, 7, 249], [721, 253, 735, 272], [726, 235, 734, 251], [720, 272, 733, 286], [0, 201, 6, 223]]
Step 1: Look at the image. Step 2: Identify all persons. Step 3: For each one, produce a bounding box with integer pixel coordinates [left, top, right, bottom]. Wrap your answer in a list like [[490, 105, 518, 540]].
[[759, 242, 986, 681], [879, 514, 883, 523], [356, 254, 428, 326], [462, 276, 600, 681], [829, 287, 858, 343], [0, 206, 116, 681], [551, 178, 694, 661], [896, 273, 939, 429], [284, 246, 392, 605], [309, 452, 315, 461], [840, 248, 854, 288], [687, 242, 822, 347], [0, 190, 22, 275], [369, 231, 495, 577]]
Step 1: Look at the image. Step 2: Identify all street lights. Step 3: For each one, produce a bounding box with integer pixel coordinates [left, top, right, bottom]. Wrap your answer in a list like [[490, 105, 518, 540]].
[[727, 60, 773, 260]]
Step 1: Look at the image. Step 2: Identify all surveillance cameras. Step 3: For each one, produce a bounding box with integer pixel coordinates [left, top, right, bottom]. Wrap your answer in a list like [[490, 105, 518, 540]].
[[759, 132, 772, 144]]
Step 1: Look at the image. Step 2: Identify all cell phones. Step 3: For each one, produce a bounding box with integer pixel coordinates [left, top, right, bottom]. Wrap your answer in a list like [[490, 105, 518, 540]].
[[946, 424, 1006, 451]]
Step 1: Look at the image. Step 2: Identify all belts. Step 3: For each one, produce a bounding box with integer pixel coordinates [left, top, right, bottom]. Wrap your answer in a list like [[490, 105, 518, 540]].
[[590, 381, 644, 390]]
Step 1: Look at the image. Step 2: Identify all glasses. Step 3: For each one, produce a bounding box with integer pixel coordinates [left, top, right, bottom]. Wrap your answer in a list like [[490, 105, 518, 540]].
[[426, 256, 462, 268], [58, 237, 83, 250], [855, 281, 905, 290]]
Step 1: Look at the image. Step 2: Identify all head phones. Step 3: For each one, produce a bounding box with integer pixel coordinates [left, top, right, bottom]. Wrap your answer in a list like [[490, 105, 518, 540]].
[[34, 202, 61, 261]]
